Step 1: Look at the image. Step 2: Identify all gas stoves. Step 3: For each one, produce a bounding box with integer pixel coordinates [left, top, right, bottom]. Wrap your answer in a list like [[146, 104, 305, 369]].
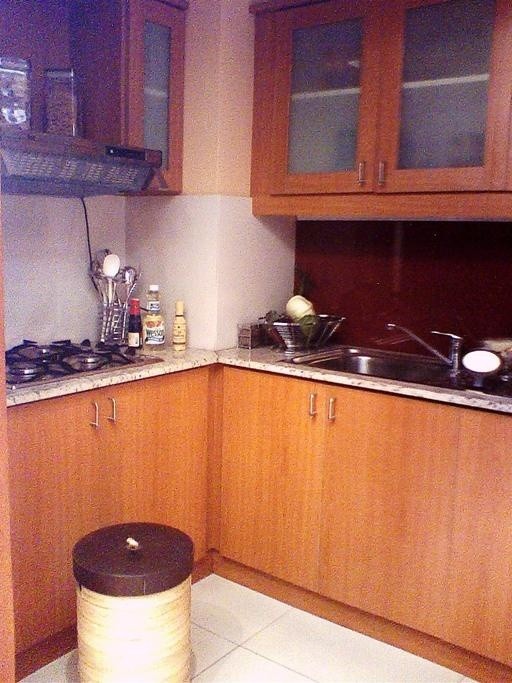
[[5, 338, 165, 390]]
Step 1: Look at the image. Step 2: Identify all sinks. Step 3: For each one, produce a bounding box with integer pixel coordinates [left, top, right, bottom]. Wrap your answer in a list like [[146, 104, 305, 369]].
[[286, 346, 446, 386]]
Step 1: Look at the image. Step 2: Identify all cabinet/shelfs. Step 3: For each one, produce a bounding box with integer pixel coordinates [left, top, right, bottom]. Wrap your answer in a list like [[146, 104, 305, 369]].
[[272, 2, 506, 196], [72, 2, 185, 197], [437, 404, 510, 665], [220, 364, 448, 642], [7, 369, 209, 648]]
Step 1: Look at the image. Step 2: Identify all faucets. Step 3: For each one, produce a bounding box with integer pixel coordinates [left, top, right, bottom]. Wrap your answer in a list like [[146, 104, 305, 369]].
[[386, 322, 462, 370]]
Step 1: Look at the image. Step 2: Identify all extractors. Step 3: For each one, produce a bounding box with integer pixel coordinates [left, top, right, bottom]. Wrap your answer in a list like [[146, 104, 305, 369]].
[[0, 126, 162, 198]]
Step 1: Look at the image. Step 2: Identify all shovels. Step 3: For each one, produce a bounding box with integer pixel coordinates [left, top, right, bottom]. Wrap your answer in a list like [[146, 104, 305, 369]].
[[103, 253, 121, 306]]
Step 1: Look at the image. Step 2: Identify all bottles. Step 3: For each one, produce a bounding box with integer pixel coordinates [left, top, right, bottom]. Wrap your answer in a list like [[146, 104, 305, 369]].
[[127, 298, 143, 351], [0, 55, 35, 142], [38, 66, 87, 140], [171, 301, 187, 351]]
